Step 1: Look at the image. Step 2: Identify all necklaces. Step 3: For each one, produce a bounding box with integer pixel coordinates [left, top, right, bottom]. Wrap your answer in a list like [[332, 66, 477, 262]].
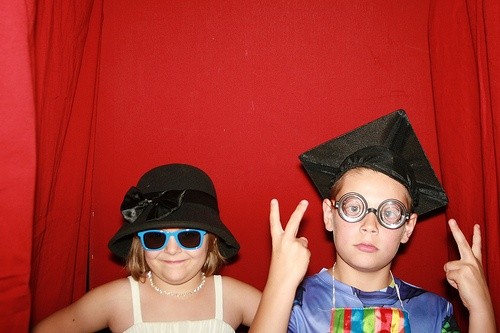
[[148, 269, 207, 296]]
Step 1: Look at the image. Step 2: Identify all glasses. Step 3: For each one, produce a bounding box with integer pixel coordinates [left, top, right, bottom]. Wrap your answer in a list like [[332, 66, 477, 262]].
[[330, 192, 410, 229], [137, 229, 207, 251]]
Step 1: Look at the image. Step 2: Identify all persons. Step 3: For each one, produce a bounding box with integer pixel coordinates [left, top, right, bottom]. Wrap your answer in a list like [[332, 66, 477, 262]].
[[28, 163, 262, 333], [244, 108, 499, 333]]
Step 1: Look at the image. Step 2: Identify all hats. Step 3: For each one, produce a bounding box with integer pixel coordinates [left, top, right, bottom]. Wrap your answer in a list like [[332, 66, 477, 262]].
[[298, 110, 449, 216], [108, 163, 240, 260]]
